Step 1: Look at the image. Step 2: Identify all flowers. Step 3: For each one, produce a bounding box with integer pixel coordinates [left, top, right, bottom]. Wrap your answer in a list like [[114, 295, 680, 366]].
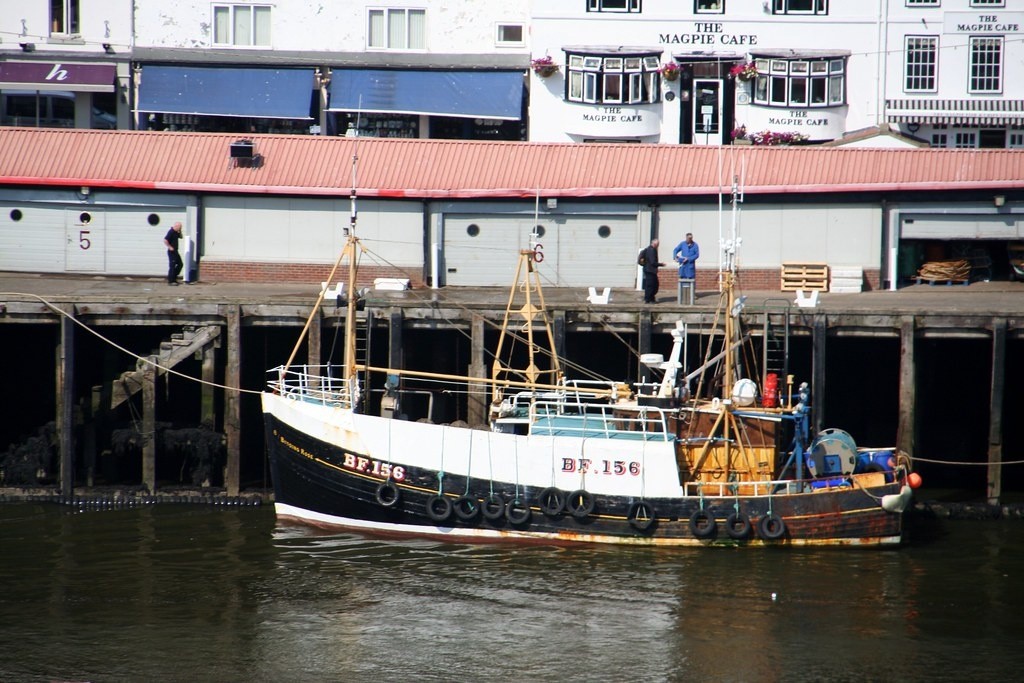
[[531, 55, 559, 74], [657, 61, 684, 79], [729, 62, 760, 80], [731, 129, 810, 146]]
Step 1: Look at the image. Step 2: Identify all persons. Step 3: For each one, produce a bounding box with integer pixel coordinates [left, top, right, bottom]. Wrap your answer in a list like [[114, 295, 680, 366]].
[[673, 233, 699, 299], [164, 222, 183, 286], [638, 238, 666, 304]]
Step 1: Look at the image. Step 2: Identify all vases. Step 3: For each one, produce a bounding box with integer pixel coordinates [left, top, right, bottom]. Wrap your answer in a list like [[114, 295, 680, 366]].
[[734, 139, 753, 146]]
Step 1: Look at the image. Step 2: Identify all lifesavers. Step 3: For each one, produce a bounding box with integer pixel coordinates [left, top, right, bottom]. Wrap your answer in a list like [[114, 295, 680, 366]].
[[566, 489, 595, 518], [481, 495, 504, 520], [628, 500, 656, 531], [376, 482, 400, 508], [537, 486, 565, 516], [689, 509, 716, 537], [453, 494, 480, 522], [757, 514, 785, 540], [425, 494, 453, 521], [726, 513, 750, 538], [505, 498, 531, 525]]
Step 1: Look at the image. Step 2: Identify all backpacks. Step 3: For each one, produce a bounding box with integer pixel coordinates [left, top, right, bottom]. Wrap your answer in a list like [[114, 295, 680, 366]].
[[638, 248, 647, 265]]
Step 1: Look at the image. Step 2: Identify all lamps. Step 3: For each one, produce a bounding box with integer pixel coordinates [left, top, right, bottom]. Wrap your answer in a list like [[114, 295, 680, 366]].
[[20, 43, 35, 51], [230, 138, 257, 159]]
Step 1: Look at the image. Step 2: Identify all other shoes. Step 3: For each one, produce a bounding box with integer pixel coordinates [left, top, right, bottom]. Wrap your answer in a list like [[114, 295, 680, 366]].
[[645, 301, 655, 304], [169, 282, 177, 285]]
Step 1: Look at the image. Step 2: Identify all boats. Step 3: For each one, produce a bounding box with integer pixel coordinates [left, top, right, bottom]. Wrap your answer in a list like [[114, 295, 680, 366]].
[[261, 94, 922, 547]]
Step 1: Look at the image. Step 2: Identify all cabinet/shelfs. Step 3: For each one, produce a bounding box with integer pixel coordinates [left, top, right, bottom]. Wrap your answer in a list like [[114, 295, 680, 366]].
[[366, 116, 413, 132]]
